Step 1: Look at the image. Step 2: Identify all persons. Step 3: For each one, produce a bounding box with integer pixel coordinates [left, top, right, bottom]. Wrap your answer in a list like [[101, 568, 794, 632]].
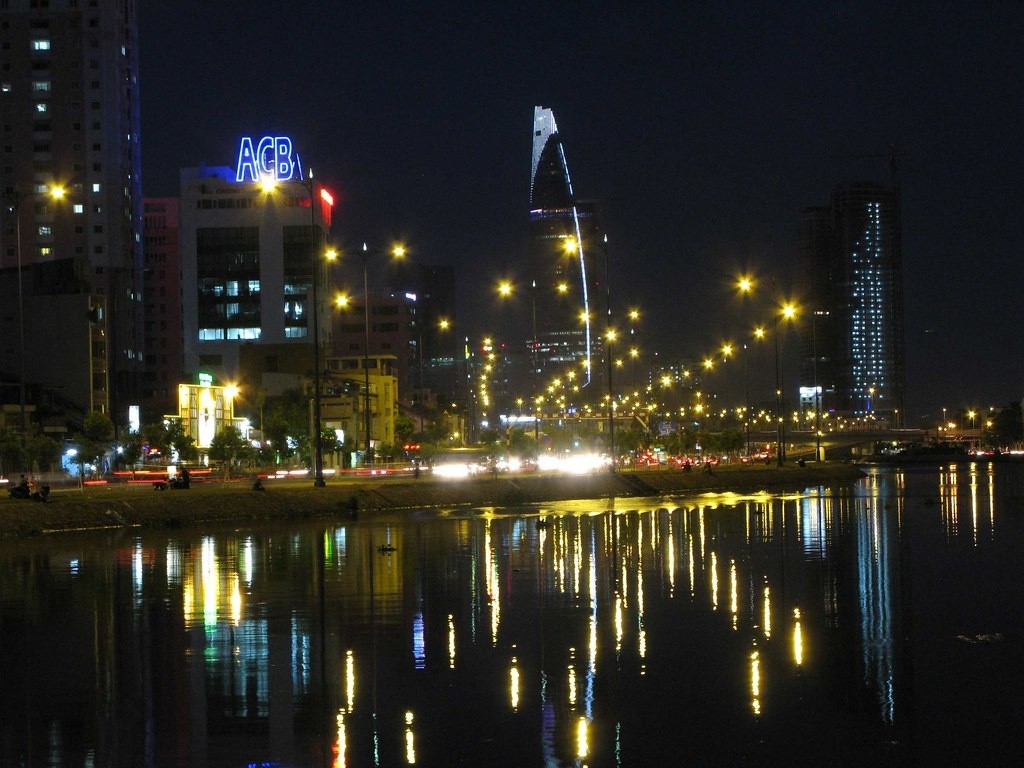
[[702, 462, 712, 475], [253, 478, 265, 491], [8, 473, 50, 502], [152, 465, 190, 490]]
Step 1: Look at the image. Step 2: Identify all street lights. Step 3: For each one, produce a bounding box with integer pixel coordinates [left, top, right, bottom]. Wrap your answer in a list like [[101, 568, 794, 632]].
[[501, 285, 538, 450], [261, 167, 326, 487], [970, 413, 974, 429], [327, 243, 404, 462], [987, 401, 993, 437], [870, 388, 874, 409], [812, 303, 830, 462], [1, 181, 63, 432], [943, 408, 946, 423], [566, 240, 616, 472], [738, 276, 783, 467], [706, 361, 728, 455], [724, 347, 749, 456], [781, 306, 793, 461], [418, 321, 447, 434], [895, 408, 898, 429]]
